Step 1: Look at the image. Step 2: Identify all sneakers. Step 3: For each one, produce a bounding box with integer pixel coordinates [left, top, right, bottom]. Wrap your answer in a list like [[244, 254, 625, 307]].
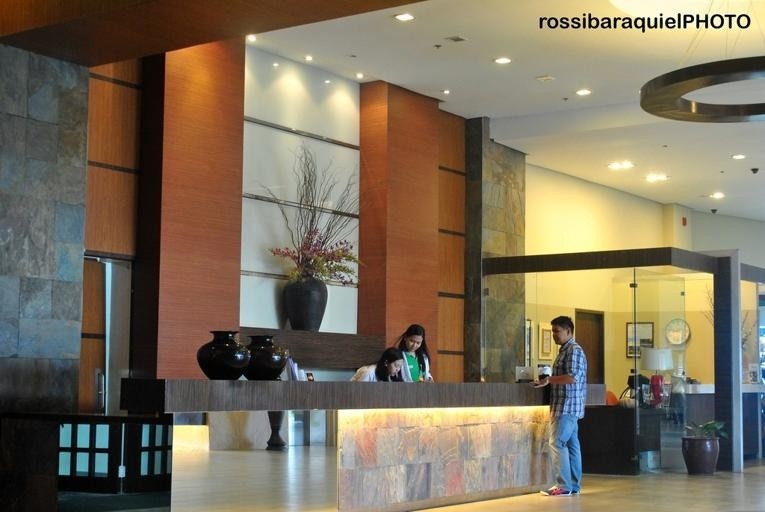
[[541, 486, 580, 496]]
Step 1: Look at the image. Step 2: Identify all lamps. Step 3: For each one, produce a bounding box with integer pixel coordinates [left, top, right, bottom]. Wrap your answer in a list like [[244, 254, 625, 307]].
[[641, 348, 673, 406]]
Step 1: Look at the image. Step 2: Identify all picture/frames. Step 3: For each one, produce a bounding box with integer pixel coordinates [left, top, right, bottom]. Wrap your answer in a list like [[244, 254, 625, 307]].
[[626, 322, 654, 358]]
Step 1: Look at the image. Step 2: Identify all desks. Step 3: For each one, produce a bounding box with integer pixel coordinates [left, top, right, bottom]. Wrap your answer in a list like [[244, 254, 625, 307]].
[[578, 404, 666, 475]]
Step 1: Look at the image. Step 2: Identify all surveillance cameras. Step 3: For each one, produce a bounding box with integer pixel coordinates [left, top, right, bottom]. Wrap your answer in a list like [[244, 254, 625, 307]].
[[752, 169, 758, 173], [711, 210, 717, 213]]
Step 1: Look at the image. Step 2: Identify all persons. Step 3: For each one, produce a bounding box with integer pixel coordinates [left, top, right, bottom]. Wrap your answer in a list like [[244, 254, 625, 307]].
[[349, 347, 404, 381], [398, 323, 435, 383], [529, 316, 587, 497]]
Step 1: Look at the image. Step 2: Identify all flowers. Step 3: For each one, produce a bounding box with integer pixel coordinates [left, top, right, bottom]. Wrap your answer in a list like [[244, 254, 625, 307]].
[[266, 230, 366, 288]]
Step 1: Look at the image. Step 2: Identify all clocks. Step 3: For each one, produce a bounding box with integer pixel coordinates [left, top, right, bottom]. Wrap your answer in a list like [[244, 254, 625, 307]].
[[665, 318, 690, 345]]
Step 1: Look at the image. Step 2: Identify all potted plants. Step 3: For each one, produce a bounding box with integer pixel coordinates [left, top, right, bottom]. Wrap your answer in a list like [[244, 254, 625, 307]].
[[681, 420, 729, 475]]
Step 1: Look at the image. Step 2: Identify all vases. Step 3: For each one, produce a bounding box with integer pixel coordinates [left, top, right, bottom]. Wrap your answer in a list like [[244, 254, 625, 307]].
[[197, 330, 287, 381], [742, 338, 749, 384], [283, 272, 327, 331]]
